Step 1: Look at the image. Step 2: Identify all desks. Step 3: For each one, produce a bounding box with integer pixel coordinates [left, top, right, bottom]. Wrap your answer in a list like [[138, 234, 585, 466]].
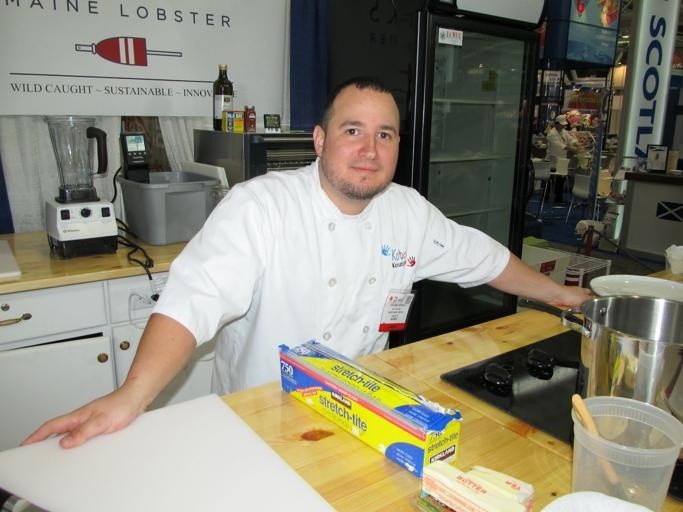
[[0, 271, 683, 511]]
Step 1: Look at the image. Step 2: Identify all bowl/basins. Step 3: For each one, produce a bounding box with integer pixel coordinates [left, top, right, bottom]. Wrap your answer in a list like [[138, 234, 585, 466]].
[[564, 391, 683, 512], [670, 169, 681, 177]]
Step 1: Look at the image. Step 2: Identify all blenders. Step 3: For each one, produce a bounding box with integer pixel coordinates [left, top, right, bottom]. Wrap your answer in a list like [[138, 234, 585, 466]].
[[46, 115, 118, 261]]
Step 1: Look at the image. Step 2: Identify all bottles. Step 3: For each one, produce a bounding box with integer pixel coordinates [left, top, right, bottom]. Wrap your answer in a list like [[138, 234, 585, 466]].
[[213, 64, 235, 132], [243, 106, 257, 136]]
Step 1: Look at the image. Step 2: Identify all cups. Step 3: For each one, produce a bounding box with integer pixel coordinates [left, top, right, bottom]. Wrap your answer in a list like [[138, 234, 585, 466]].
[[664, 244, 683, 276]]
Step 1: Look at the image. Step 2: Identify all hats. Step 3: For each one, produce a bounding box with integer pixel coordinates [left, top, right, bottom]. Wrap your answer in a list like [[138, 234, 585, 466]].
[[556, 115, 568, 125]]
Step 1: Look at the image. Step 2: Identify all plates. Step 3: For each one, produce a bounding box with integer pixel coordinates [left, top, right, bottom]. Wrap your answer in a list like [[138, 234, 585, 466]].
[[590, 273, 682, 304]]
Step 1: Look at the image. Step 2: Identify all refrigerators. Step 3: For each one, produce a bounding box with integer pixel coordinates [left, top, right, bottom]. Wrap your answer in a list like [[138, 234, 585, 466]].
[[287, 1, 541, 343]]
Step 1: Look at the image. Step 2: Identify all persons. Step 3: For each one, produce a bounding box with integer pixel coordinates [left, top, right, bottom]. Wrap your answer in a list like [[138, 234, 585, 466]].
[[20, 77, 600, 449], [541, 115, 578, 206]]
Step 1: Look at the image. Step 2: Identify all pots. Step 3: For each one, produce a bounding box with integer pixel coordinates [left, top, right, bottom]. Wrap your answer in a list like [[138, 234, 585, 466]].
[[559, 293, 683, 463]]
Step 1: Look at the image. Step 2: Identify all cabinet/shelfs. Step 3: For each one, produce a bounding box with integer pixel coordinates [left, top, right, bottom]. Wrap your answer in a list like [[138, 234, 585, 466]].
[[0, 270, 214, 452]]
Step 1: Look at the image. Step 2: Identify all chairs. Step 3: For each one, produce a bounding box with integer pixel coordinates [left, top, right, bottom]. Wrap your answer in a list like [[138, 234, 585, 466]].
[[530, 157, 613, 224]]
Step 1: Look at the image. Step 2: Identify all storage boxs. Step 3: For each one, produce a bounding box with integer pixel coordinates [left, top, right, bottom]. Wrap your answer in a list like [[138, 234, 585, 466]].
[[118, 171, 221, 245]]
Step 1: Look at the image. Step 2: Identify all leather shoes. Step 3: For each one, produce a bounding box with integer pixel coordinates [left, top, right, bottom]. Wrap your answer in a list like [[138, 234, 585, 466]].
[[554, 201, 568, 206]]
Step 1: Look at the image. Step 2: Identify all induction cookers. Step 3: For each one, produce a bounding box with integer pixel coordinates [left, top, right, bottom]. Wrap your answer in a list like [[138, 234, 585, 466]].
[[443, 325, 683, 501]]
